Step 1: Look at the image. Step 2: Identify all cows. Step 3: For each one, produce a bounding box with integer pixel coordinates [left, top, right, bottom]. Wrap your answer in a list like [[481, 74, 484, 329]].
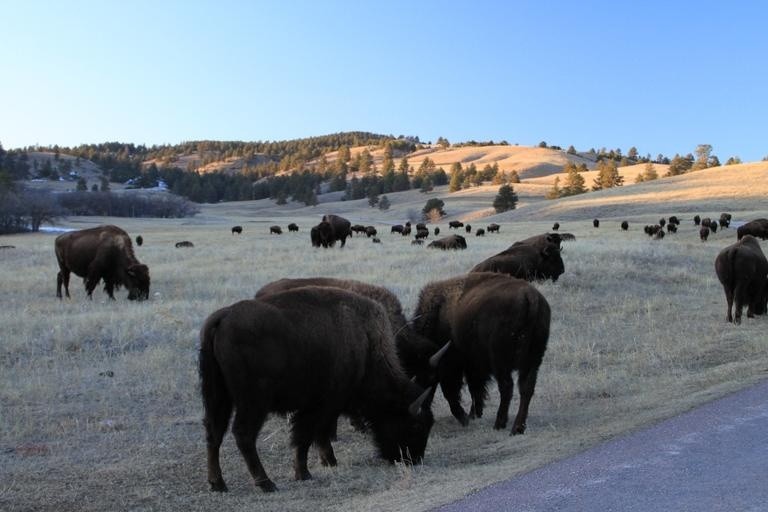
[[487, 223, 500, 233], [197, 285, 435, 492], [231, 225, 243, 235], [714, 236, 768, 325], [255, 277, 452, 467], [411, 271, 551, 435], [310, 213, 473, 249], [269, 225, 283, 236], [550, 213, 768, 241], [53, 224, 151, 303], [468, 242, 564, 282], [176, 241, 194, 248], [511, 233, 563, 247], [287, 223, 299, 232], [475, 228, 485, 237]]
